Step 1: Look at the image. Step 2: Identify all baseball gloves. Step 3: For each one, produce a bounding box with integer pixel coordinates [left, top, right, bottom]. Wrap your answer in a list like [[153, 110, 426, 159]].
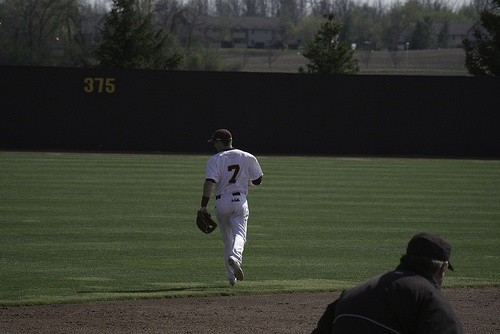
[[195, 208, 218, 235]]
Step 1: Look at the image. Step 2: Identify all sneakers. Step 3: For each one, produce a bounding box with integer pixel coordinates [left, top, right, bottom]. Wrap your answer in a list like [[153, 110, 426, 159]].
[[229, 280, 235, 286], [227, 255, 244, 281]]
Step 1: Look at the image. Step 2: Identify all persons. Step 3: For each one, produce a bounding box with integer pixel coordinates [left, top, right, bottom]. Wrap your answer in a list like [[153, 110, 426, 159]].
[[196, 129, 263, 286], [310, 232, 464, 334]]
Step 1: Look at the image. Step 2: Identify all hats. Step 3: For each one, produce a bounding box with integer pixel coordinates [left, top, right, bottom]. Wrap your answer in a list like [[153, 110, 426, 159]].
[[407, 231, 455, 272], [208, 129, 232, 143]]
